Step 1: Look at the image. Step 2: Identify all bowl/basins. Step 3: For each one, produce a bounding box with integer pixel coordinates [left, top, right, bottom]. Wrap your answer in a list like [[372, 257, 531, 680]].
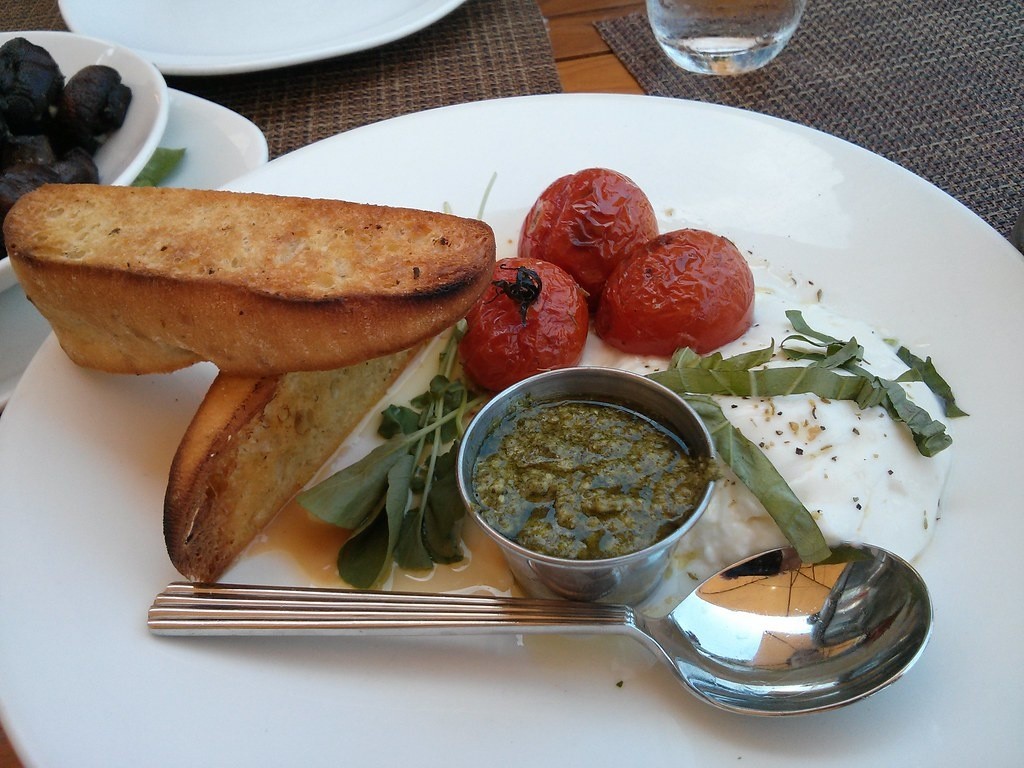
[[0, 27, 166, 298]]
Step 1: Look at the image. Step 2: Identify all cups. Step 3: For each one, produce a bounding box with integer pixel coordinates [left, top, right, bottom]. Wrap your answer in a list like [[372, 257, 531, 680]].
[[455, 366, 720, 607], [647, 1, 803, 78]]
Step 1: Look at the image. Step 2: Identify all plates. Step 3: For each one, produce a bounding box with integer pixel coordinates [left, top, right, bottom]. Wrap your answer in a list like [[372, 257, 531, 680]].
[[58, 0, 464, 75], [0, 87, 269, 407], [0, 90, 1022, 768]]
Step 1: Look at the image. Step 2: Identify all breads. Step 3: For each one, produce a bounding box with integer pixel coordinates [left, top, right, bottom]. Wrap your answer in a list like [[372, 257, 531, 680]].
[[1, 183, 498, 590]]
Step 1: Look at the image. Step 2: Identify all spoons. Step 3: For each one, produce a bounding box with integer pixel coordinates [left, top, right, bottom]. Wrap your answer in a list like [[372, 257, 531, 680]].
[[148, 533, 934, 718]]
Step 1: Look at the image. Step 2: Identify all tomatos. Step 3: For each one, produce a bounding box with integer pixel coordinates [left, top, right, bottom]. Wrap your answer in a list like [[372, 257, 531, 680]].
[[460, 168, 755, 392]]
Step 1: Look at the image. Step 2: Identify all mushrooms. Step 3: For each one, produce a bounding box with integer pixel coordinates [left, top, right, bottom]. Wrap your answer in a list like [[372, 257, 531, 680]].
[[0, 38, 133, 215]]
